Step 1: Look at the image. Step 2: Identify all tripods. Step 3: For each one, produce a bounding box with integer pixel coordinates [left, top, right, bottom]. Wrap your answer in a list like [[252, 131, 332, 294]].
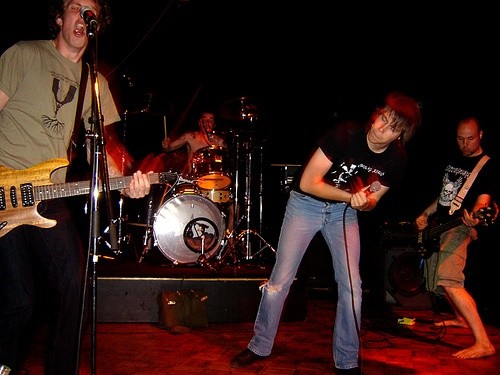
[[216, 116, 277, 266], [98, 106, 137, 256]]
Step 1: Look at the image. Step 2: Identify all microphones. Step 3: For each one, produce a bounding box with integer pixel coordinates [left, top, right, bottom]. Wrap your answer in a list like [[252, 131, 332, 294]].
[[79, 5, 98, 28], [365, 181, 382, 196]]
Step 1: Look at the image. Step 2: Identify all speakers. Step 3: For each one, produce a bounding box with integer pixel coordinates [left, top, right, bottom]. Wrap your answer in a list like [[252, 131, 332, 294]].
[[380, 245, 438, 311]]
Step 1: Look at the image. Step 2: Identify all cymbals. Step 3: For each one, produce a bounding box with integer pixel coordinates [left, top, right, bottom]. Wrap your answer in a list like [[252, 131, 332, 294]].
[[208, 129, 256, 135], [222, 97, 263, 119]]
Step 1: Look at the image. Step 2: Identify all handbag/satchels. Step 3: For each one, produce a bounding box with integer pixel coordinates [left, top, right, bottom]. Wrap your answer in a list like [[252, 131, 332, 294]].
[[160, 289, 209, 333]]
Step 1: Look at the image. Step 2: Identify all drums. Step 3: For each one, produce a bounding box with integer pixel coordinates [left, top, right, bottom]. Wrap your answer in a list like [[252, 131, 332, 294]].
[[195, 144, 231, 191], [153, 184, 226, 265], [197, 188, 233, 204]]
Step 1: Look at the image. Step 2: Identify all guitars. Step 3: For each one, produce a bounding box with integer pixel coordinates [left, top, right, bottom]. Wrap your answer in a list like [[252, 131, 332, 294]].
[[411, 203, 500, 260], [0, 157, 198, 240]]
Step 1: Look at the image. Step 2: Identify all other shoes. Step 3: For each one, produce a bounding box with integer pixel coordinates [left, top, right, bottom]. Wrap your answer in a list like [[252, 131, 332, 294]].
[[236, 348, 268, 364], [333, 366, 364, 375]]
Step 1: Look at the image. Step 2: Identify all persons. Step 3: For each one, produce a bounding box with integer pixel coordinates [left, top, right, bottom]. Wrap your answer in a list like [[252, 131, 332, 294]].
[[231, 93, 421, 375], [416, 114, 500, 359], [0, 0, 150, 375], [162, 110, 229, 182]]
